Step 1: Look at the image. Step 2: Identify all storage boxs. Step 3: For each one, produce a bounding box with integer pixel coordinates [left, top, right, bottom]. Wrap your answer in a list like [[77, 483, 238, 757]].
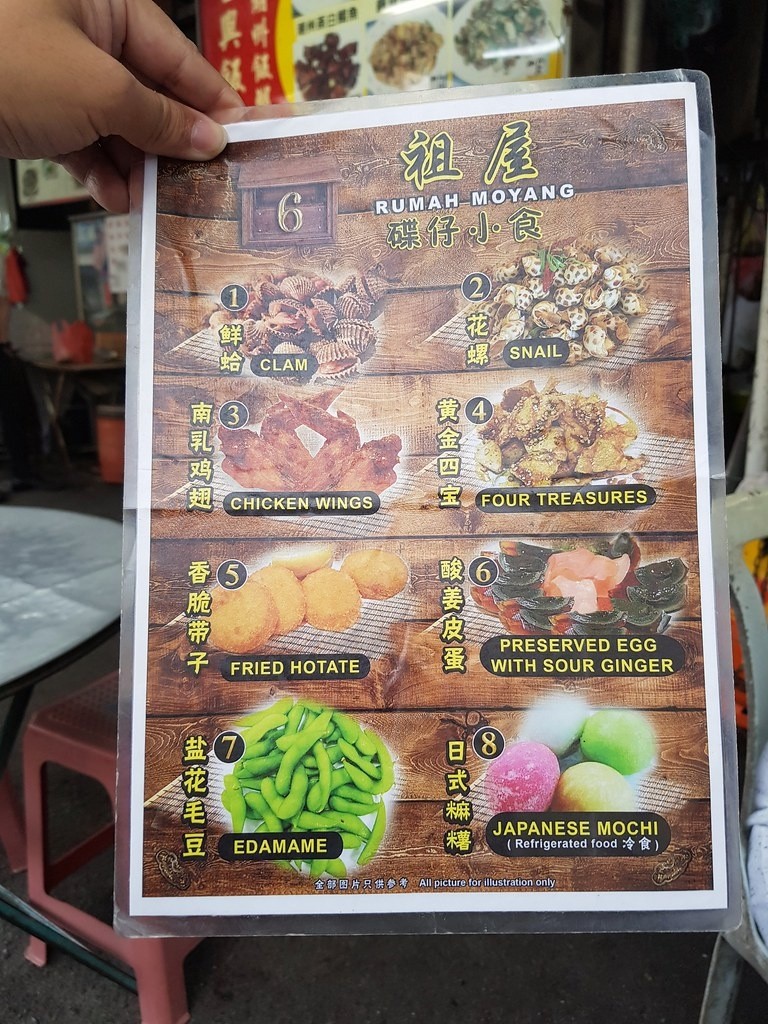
[[94, 332, 126, 359]]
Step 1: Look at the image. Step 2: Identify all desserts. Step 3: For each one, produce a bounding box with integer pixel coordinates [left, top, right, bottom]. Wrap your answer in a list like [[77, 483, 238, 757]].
[[484, 689, 654, 809]]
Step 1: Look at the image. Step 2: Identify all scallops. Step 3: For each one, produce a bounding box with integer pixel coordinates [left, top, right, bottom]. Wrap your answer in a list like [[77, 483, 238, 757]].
[[213, 268, 390, 385]]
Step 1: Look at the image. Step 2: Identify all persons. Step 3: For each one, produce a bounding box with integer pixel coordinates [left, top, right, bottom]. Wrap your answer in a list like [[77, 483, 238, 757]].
[[0, 1, 245, 218]]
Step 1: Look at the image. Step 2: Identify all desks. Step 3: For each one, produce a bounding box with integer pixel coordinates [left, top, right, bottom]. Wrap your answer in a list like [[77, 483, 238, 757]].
[[18, 350, 123, 485], [1, 505, 219, 1006]]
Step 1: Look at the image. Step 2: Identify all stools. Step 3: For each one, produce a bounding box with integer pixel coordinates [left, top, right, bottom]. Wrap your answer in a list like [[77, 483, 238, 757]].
[[23, 668, 203, 1022]]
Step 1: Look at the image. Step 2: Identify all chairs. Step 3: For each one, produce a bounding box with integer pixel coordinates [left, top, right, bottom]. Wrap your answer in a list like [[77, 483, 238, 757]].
[[697, 489, 768, 1023]]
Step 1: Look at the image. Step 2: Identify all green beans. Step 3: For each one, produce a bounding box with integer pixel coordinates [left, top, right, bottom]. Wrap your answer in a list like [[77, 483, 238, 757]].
[[217, 698, 395, 880]]
